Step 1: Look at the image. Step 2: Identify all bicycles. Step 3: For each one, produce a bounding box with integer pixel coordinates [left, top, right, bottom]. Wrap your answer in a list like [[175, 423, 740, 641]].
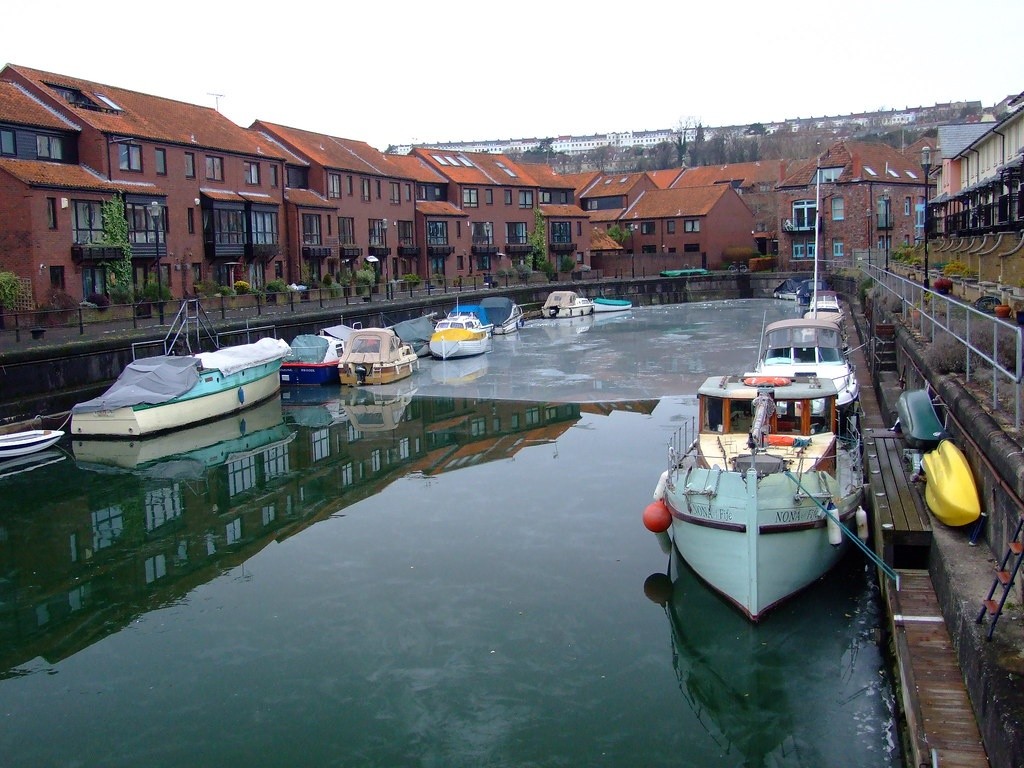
[[728, 261, 748, 273]]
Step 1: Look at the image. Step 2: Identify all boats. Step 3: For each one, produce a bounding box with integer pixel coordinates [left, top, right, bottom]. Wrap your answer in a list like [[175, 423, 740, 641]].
[[541, 291, 632, 319], [279, 321, 420, 387], [642, 376, 866, 619], [0, 430, 65, 457], [895, 388, 948, 450], [386, 297, 524, 360], [71, 338, 290, 436], [774, 278, 848, 357], [920, 440, 980, 526], [741, 158, 859, 434], [643, 532, 866, 768], [71, 389, 296, 481]]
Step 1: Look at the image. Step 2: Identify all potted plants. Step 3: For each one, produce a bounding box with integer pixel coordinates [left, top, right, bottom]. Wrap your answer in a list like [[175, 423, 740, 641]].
[[453, 279, 461, 287]]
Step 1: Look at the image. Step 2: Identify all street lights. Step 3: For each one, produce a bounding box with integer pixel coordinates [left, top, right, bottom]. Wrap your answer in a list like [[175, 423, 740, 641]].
[[486, 221, 490, 283], [921, 145, 932, 305], [630, 224, 635, 278], [143, 202, 166, 325], [382, 218, 389, 299], [883, 189, 890, 275], [866, 208, 871, 270]]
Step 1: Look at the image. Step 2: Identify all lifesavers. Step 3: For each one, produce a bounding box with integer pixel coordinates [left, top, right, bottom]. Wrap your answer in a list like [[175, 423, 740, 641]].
[[740, 265, 747, 272], [744, 377, 791, 387], [728, 265, 737, 273], [974, 296, 1001, 314]]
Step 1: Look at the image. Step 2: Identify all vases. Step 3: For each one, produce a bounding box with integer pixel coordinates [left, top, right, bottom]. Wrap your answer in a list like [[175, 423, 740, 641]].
[[994, 304, 1011, 318], [937, 287, 948, 294]]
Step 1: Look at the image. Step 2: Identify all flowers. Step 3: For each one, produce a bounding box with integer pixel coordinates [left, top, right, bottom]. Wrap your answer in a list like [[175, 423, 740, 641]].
[[934, 277, 952, 289]]
